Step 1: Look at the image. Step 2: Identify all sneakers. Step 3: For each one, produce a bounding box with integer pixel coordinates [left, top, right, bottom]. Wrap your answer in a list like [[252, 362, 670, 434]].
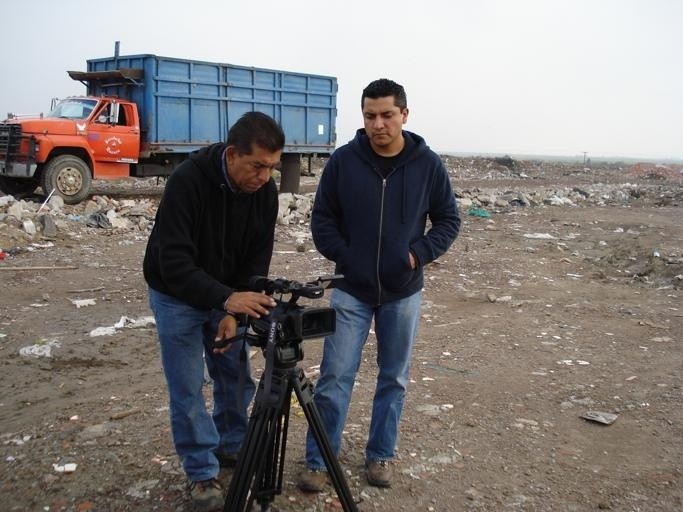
[[215, 452, 256, 470], [365, 453, 394, 486], [186, 475, 226, 512], [299, 463, 329, 492]]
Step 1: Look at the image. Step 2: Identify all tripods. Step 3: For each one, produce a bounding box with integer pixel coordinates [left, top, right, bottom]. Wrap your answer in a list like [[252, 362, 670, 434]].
[[224, 369, 361, 511]]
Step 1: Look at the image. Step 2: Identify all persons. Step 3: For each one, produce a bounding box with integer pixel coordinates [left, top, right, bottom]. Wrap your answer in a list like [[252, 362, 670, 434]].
[[141, 111, 285, 512], [295, 80, 462, 495]]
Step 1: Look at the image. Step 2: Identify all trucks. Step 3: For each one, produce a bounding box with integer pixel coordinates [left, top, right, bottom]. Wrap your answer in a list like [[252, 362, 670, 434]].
[[1, 39, 339, 203]]
